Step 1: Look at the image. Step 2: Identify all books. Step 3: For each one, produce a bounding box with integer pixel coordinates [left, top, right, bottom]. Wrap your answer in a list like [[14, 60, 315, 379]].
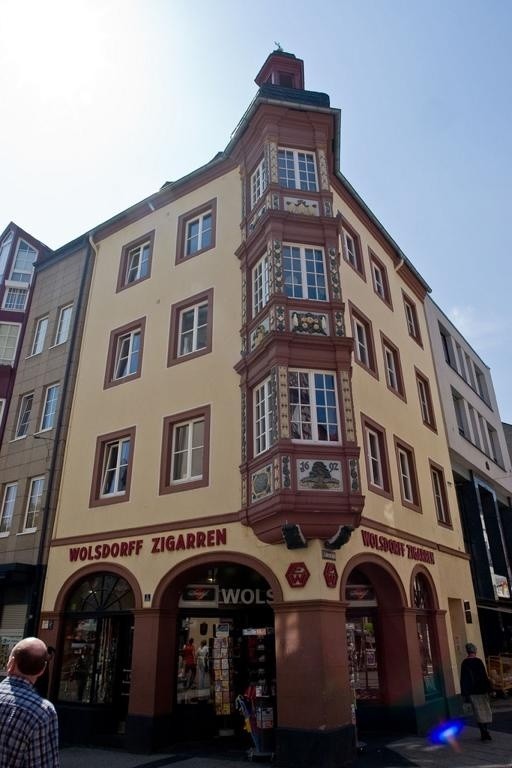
[[212, 623, 234, 718]]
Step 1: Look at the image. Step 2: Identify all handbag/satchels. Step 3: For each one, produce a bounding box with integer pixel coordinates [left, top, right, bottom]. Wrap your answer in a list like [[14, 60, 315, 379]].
[[458, 702, 474, 717]]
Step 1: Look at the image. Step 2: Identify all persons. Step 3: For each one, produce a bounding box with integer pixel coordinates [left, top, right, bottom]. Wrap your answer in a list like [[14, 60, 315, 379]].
[[417, 632, 432, 694], [0, 636, 60, 768], [181, 637, 209, 689], [69, 644, 91, 705], [460, 643, 493, 742]]
